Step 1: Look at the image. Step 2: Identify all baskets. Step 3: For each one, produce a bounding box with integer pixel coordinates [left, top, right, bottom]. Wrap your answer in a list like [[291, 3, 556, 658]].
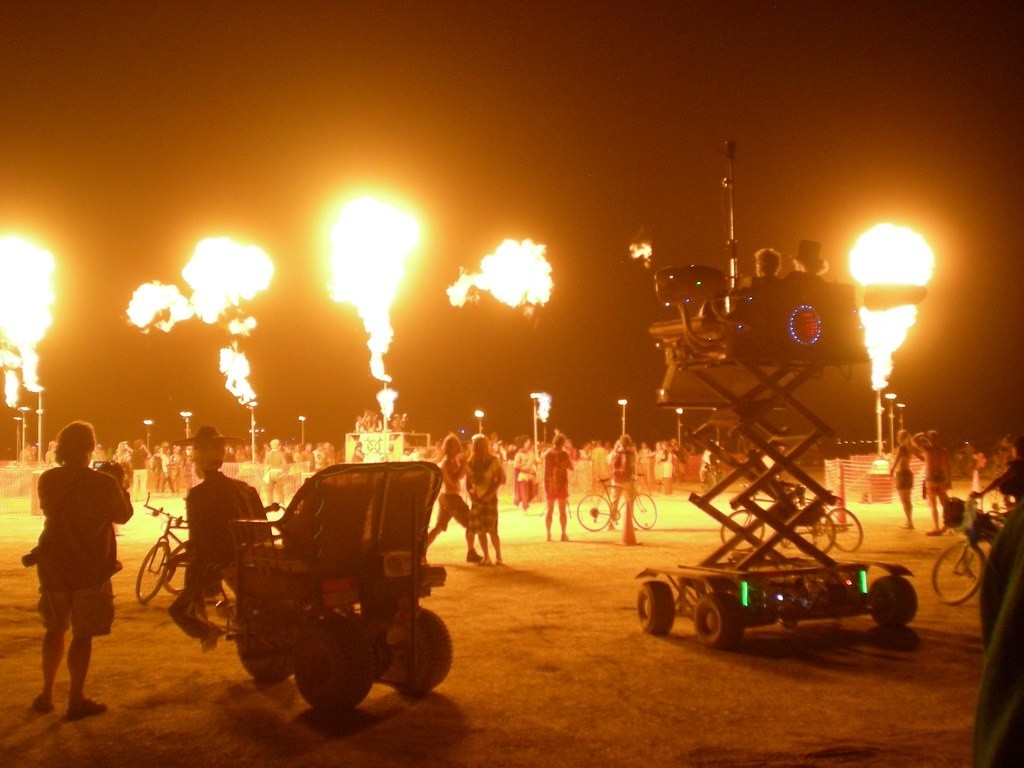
[[942, 495, 963, 528]]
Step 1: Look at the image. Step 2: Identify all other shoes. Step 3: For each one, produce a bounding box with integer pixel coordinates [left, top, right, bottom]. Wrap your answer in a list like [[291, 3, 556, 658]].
[[466, 549, 482, 560], [33, 695, 55, 712], [67, 698, 108, 719]]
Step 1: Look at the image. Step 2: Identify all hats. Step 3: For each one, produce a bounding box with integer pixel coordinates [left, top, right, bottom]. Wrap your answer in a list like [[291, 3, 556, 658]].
[[171, 425, 244, 447]]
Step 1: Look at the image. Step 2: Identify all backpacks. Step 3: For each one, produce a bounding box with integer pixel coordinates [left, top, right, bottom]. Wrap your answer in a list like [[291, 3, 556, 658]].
[[613, 449, 631, 483]]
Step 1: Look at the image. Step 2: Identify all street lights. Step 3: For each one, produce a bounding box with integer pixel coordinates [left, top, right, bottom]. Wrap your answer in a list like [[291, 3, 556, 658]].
[[179, 411, 192, 439], [618, 399, 627, 436], [474, 410, 485, 434], [142, 419, 154, 451], [298, 415, 306, 444]]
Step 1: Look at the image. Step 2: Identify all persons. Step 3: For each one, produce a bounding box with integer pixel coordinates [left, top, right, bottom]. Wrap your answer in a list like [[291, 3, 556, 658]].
[[168, 425, 272, 653], [542, 435, 574, 541], [973, 436, 1024, 503], [427, 433, 484, 562], [351, 442, 365, 462], [20, 445, 38, 462], [640, 437, 686, 495], [890, 429, 924, 530], [466, 433, 505, 566], [755, 248, 782, 277], [402, 441, 444, 463], [514, 435, 540, 514], [44, 440, 56, 462], [225, 442, 335, 472], [265, 439, 287, 505], [608, 435, 639, 530], [971, 501, 1024, 768], [788, 239, 827, 275], [566, 439, 612, 478], [912, 430, 952, 536], [31, 420, 133, 720], [92, 439, 193, 495], [490, 433, 503, 453], [699, 449, 722, 487]]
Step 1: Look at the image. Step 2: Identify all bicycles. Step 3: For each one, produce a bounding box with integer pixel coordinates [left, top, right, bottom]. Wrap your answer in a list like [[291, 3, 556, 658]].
[[134, 491, 188, 606], [929, 492, 1016, 607], [576, 472, 658, 533], [718, 475, 864, 559]]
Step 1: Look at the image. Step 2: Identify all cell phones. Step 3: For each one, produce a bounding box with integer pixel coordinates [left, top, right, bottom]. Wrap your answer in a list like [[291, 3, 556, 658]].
[[92, 461, 104, 468]]
